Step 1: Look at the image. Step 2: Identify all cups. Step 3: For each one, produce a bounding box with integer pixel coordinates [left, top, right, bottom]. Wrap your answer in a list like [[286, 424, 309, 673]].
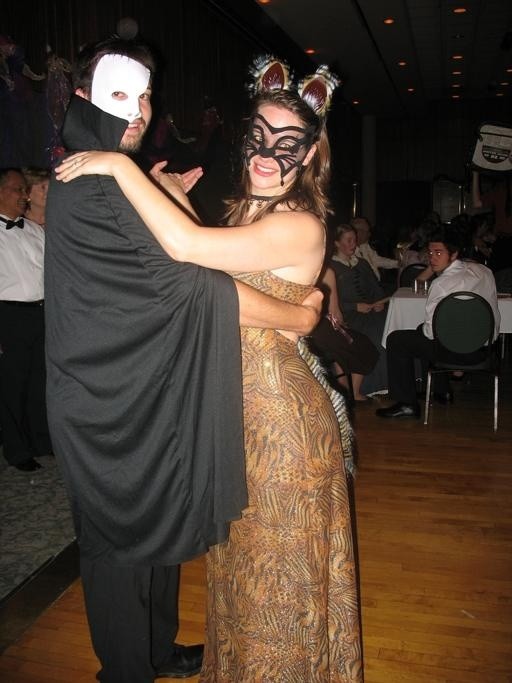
[[412, 278, 429, 296]]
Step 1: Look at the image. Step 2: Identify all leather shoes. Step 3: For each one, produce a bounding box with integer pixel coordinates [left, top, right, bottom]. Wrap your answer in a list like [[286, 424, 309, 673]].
[[4, 453, 37, 471], [154, 643, 205, 679], [376, 401, 420, 420], [433, 390, 454, 402], [352, 396, 374, 407]]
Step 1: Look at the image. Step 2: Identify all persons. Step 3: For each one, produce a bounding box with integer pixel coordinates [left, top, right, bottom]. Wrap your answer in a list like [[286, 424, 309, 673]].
[[44, 37, 326, 682], [53, 61, 368, 683], [0, 167, 49, 472], [377, 230, 501, 419], [20, 169, 49, 228], [305, 210, 399, 404], [393, 213, 512, 296]]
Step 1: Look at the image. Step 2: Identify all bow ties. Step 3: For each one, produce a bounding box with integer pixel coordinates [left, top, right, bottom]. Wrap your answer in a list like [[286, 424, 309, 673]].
[[0, 216, 24, 230]]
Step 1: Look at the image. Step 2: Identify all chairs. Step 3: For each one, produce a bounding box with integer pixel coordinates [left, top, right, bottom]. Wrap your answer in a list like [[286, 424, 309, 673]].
[[398, 262, 437, 288], [423, 291, 501, 431]]
